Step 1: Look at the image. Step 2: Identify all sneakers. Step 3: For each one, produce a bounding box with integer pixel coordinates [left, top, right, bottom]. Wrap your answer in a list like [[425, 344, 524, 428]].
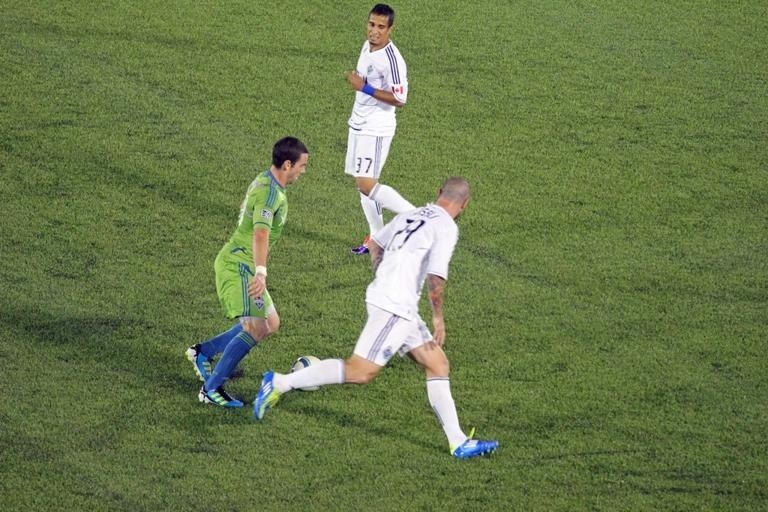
[[352, 236, 385, 255], [184, 342, 213, 382], [254, 370, 282, 420], [198, 383, 244, 408], [449, 426, 499, 459]]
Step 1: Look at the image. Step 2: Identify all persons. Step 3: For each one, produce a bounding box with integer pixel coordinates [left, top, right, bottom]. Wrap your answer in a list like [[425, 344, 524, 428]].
[[250, 175, 501, 462], [342, 3, 421, 255], [180, 135, 310, 412]]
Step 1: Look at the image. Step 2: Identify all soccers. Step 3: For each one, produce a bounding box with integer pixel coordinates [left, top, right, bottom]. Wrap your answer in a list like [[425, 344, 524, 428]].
[[291, 355, 323, 392]]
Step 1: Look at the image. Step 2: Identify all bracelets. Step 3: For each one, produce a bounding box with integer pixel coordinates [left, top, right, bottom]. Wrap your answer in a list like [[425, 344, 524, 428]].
[[254, 264, 269, 278], [362, 83, 377, 100]]
